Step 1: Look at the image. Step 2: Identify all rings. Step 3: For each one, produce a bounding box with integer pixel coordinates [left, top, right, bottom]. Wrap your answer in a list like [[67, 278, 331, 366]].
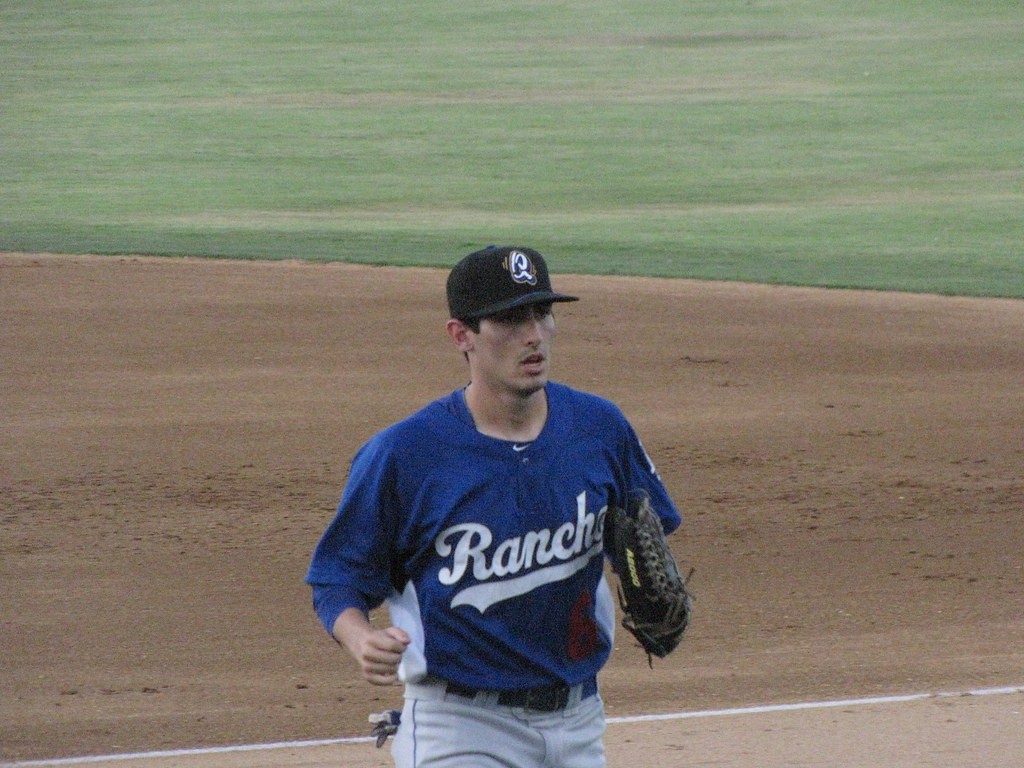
[[386, 662, 395, 674]]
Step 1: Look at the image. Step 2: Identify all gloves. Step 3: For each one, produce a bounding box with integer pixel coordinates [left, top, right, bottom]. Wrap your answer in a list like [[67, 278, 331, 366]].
[[369, 708, 399, 748]]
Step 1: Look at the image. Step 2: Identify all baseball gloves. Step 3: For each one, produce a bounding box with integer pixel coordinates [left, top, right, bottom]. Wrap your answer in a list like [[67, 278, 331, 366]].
[[603, 498, 694, 660]]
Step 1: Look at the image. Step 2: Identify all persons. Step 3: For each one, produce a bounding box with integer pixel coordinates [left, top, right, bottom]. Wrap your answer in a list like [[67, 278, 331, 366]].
[[302, 239, 696, 768]]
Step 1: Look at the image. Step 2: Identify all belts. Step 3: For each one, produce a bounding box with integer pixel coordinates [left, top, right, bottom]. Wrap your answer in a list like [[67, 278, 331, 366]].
[[445, 676, 597, 713]]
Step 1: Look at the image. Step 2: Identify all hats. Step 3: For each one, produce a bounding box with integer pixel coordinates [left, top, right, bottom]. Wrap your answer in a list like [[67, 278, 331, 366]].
[[446, 244, 579, 318]]
